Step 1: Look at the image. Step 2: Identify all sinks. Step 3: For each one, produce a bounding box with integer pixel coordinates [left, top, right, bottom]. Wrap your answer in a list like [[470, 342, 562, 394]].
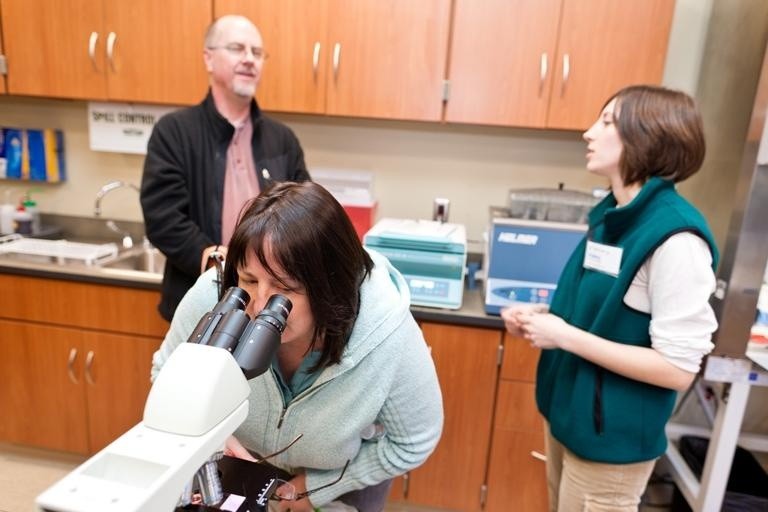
[[100, 248, 165, 275]]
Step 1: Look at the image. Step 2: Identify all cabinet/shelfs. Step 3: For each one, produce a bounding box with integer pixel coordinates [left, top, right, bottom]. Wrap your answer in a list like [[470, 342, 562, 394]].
[[483, 326, 552, 512], [383, 321, 502, 512], [0, 269, 170, 457]]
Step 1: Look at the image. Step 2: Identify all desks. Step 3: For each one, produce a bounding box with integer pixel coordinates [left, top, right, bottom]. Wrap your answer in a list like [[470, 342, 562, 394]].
[[658, 353, 768, 512]]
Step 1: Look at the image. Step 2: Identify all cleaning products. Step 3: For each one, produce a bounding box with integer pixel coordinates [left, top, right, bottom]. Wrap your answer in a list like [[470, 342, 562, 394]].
[[13, 186, 42, 237]]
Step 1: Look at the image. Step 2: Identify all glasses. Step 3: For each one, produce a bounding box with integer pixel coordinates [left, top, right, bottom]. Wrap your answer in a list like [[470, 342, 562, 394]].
[[245, 433, 352, 505]]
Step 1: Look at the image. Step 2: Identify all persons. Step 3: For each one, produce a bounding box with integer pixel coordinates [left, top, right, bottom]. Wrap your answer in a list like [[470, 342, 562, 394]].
[[147, 182, 444, 512], [500, 86, 721, 512], [139, 13, 311, 324]]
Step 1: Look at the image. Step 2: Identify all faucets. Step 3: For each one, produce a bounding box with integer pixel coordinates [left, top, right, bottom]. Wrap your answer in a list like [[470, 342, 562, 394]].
[[94, 180, 143, 220]]
